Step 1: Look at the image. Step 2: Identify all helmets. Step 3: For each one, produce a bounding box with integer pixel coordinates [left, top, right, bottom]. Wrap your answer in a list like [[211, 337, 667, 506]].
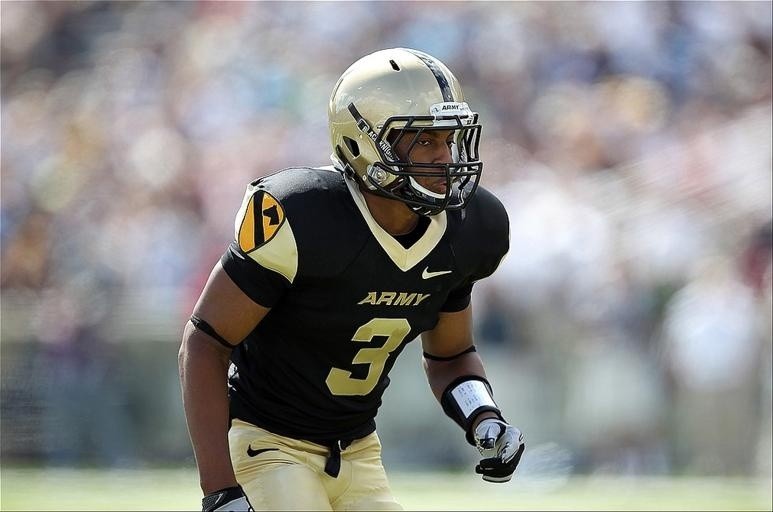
[[326, 44, 475, 193]]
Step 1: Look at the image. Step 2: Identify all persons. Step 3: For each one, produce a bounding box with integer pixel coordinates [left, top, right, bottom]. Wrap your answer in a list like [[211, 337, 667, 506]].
[[175, 46, 526, 512]]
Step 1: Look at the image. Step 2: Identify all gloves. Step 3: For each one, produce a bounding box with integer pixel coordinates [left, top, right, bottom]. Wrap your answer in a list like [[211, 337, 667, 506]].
[[472, 417, 527, 484], [200, 482, 254, 511]]
